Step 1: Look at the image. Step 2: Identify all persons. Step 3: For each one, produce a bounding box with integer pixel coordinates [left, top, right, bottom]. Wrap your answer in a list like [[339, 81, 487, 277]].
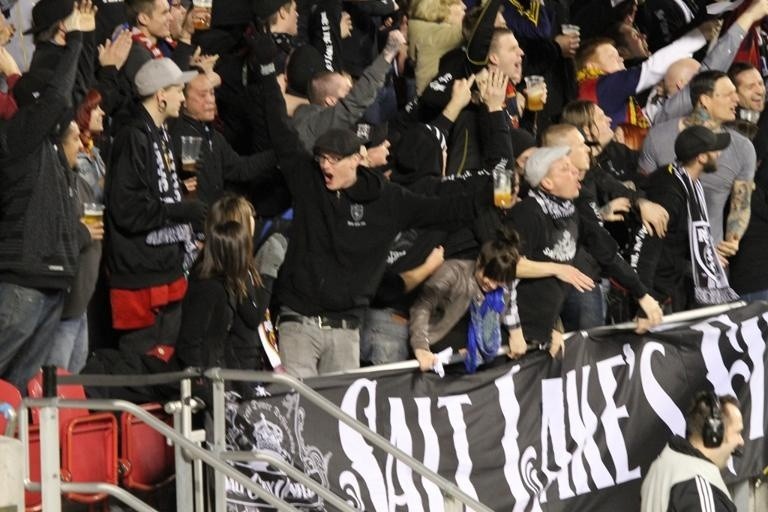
[[0, 1, 768, 403], [640, 392, 745, 511]]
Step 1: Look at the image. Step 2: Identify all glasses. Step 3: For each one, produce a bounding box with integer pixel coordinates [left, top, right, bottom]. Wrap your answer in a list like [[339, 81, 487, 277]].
[[314, 153, 345, 164]]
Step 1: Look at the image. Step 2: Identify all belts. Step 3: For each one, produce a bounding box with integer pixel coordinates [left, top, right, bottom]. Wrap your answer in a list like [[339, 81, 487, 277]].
[[277, 313, 361, 330]]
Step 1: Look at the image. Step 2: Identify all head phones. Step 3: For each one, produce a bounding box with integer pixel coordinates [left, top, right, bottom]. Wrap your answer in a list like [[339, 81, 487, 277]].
[[703, 391, 723, 447]]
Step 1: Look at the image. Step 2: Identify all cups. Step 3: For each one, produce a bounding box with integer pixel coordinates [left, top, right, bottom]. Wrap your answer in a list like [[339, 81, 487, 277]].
[[180, 136, 203, 171], [83, 203, 105, 224], [193, 0, 211, 30], [524, 75, 545, 111], [561, 24, 580, 36], [492, 169, 512, 209]]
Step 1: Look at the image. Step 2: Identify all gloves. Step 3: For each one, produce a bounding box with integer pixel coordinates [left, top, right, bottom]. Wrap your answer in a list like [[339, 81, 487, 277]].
[[166, 200, 209, 227]]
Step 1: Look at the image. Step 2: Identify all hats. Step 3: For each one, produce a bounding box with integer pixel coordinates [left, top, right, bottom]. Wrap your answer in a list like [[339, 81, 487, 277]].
[[350, 121, 386, 148], [134, 57, 199, 96], [22, 1, 72, 34], [525, 145, 570, 189], [312, 129, 360, 156], [674, 126, 730, 158]]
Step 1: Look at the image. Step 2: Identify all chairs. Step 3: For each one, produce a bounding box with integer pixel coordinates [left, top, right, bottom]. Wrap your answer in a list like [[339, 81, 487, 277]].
[[0, 368, 176, 512]]
